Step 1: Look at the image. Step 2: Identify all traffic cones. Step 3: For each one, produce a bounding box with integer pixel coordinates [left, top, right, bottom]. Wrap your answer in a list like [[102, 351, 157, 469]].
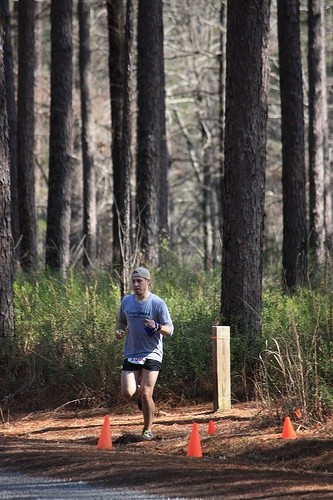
[[277, 415, 299, 440], [207, 420, 217, 435], [95, 415, 113, 450], [184, 422, 204, 459]]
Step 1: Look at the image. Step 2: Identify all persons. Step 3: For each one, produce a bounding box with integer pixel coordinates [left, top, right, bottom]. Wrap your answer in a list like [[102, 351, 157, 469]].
[[114, 267, 174, 439]]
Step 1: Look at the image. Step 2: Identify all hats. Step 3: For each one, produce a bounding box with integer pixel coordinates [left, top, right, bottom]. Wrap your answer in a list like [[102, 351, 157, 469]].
[[131, 267, 150, 279]]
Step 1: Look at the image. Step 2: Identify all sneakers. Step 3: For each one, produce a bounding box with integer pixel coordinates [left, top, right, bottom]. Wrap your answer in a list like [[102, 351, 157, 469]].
[[137, 395, 143, 411], [141, 430, 152, 441]]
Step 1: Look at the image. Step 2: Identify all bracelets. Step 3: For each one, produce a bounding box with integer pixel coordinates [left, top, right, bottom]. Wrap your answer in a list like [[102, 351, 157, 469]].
[[157, 324, 162, 331]]
